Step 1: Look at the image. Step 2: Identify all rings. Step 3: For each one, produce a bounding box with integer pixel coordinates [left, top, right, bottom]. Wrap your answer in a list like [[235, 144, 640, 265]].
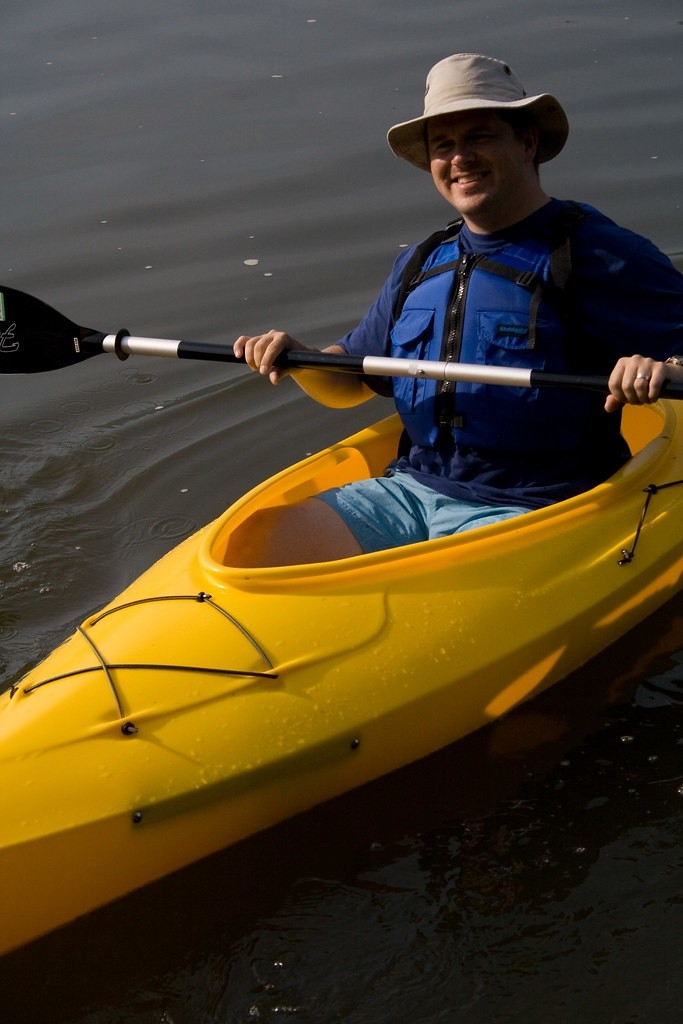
[[635, 374, 649, 380]]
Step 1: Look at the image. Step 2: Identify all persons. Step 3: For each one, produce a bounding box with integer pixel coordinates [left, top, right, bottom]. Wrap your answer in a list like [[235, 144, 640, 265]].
[[224, 52, 683, 594]]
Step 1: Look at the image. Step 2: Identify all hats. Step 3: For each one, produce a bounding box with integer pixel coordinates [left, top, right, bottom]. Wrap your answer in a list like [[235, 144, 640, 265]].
[[387, 53, 569, 173]]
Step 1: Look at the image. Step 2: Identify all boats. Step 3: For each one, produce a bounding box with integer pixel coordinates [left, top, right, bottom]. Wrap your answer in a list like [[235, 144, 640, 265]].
[[0, 370, 682, 955]]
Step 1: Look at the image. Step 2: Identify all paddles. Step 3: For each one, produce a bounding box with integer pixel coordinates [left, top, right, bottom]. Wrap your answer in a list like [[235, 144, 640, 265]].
[[0, 282, 682, 400]]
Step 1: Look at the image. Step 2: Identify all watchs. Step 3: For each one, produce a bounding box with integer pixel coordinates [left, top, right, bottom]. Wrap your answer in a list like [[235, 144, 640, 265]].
[[666, 355, 683, 364]]
